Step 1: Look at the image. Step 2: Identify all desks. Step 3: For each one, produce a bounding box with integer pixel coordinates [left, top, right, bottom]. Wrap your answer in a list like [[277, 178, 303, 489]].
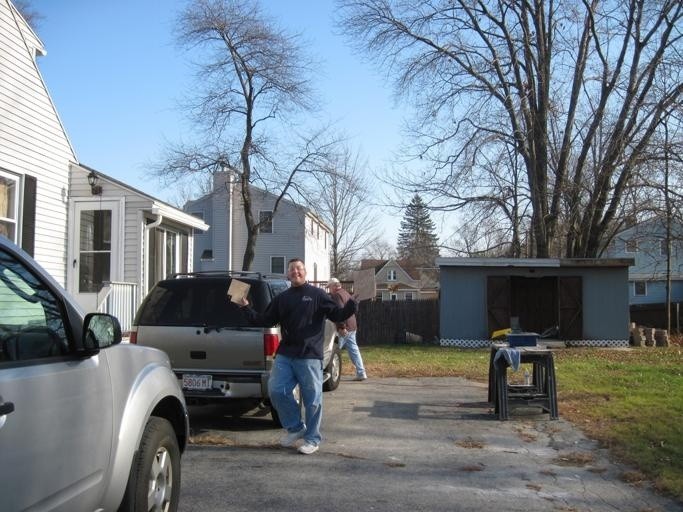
[[488, 342, 558, 421]]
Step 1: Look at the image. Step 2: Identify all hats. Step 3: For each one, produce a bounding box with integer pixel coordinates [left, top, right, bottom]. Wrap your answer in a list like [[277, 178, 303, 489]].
[[324, 278, 340, 288]]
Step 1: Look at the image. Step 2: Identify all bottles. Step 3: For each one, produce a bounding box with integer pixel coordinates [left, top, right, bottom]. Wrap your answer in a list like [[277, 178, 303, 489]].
[[524, 367, 530, 384], [339, 331, 348, 349]]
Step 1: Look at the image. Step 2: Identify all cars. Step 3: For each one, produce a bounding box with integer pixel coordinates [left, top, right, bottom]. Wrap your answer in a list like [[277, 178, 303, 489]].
[[0, 233, 188, 512]]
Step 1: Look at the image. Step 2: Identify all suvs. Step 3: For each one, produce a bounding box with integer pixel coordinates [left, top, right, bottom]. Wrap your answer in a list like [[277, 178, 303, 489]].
[[129, 270, 342, 428]]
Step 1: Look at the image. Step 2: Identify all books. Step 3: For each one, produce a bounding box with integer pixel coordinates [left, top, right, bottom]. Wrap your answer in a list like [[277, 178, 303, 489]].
[[224, 277, 251, 306]]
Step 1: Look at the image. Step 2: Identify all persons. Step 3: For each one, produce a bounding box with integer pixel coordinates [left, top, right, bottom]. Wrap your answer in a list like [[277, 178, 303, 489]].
[[238, 257, 361, 455], [323, 276, 368, 382]]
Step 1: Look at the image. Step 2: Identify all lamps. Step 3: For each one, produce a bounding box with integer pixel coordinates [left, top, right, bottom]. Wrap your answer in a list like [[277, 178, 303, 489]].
[[87, 171, 102, 195]]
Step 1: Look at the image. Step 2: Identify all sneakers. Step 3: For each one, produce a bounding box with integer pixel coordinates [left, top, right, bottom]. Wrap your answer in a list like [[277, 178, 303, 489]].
[[279, 424, 318, 455], [352, 372, 368, 380]]
